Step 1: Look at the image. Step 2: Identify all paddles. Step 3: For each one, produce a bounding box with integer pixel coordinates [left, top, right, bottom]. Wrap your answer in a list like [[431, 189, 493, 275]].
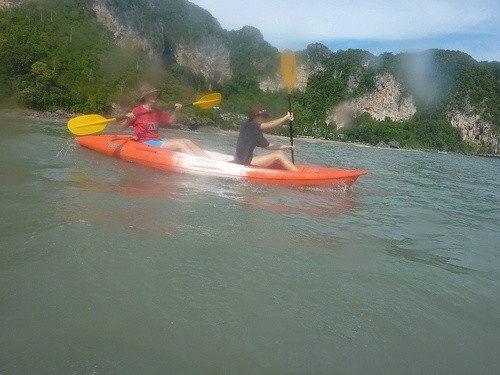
[[67, 93, 222, 136], [281, 49, 297, 164]]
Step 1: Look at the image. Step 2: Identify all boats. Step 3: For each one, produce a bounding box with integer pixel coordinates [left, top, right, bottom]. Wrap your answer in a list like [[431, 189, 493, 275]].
[[73, 133, 368, 190]]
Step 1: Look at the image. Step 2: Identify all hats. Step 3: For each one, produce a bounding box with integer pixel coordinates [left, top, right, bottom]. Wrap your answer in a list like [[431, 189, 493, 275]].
[[247, 106, 272, 123], [137, 84, 161, 103]]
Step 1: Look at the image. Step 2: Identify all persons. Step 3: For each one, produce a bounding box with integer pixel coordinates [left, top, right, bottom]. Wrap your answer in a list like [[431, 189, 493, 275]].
[[234, 105, 301, 171], [121, 85, 203, 155]]
